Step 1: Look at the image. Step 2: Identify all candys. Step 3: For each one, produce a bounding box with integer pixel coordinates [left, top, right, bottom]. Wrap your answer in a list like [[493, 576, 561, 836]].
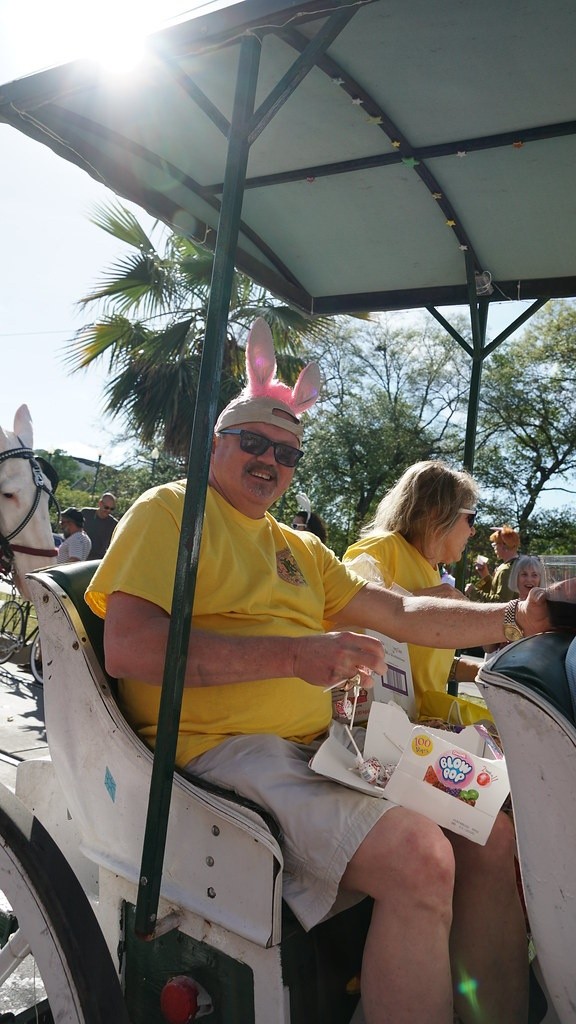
[[323, 661, 405, 789]]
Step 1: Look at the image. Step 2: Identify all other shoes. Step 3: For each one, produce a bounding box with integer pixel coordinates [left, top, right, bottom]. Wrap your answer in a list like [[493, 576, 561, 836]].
[[18, 658, 42, 673]]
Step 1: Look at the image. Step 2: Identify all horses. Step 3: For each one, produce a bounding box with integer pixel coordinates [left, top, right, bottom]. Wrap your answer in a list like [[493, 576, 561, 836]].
[[0, 402, 57, 602]]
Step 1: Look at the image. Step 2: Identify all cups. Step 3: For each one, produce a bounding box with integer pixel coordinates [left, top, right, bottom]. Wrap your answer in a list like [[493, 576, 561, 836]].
[[475, 555, 489, 570], [537, 554, 576, 641]]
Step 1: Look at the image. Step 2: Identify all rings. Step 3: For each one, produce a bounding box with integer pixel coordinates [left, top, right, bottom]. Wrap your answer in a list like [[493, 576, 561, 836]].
[[349, 674, 360, 685]]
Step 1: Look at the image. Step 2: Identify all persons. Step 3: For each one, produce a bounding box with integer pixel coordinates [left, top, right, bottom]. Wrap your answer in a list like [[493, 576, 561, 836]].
[[83, 401, 576, 1024], [80, 492, 120, 561], [290, 511, 326, 546], [57, 507, 91, 565], [343, 460, 501, 765], [465, 525, 520, 603], [509, 555, 546, 601]]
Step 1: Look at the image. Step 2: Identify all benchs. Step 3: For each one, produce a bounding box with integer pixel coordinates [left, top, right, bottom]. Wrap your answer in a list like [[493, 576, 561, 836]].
[[20, 557, 576, 1024]]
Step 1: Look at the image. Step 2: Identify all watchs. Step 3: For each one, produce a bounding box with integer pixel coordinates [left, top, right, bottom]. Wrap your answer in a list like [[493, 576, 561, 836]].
[[504, 599, 523, 642]]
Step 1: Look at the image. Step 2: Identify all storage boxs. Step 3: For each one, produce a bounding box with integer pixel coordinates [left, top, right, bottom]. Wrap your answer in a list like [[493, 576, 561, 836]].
[[306, 701, 511, 846]]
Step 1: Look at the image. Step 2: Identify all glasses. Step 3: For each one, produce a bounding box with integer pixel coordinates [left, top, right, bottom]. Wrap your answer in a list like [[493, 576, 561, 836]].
[[219, 430, 304, 467], [101, 501, 115, 510], [492, 543, 499, 548], [459, 508, 477, 528], [291, 523, 307, 529]]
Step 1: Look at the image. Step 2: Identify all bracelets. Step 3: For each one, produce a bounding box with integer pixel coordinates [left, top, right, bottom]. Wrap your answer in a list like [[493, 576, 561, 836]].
[[449, 656, 461, 683]]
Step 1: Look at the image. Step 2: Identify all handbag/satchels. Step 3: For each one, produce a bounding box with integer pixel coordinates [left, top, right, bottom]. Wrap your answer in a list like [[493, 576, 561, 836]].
[[418, 690, 503, 754]]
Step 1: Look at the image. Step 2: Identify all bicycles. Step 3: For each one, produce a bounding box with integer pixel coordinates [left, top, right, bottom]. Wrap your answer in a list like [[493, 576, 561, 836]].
[[0, 593, 46, 686]]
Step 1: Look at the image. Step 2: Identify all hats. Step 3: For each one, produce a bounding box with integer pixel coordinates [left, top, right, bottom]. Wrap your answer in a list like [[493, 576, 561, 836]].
[[57, 507, 85, 522], [214, 395, 304, 450]]
